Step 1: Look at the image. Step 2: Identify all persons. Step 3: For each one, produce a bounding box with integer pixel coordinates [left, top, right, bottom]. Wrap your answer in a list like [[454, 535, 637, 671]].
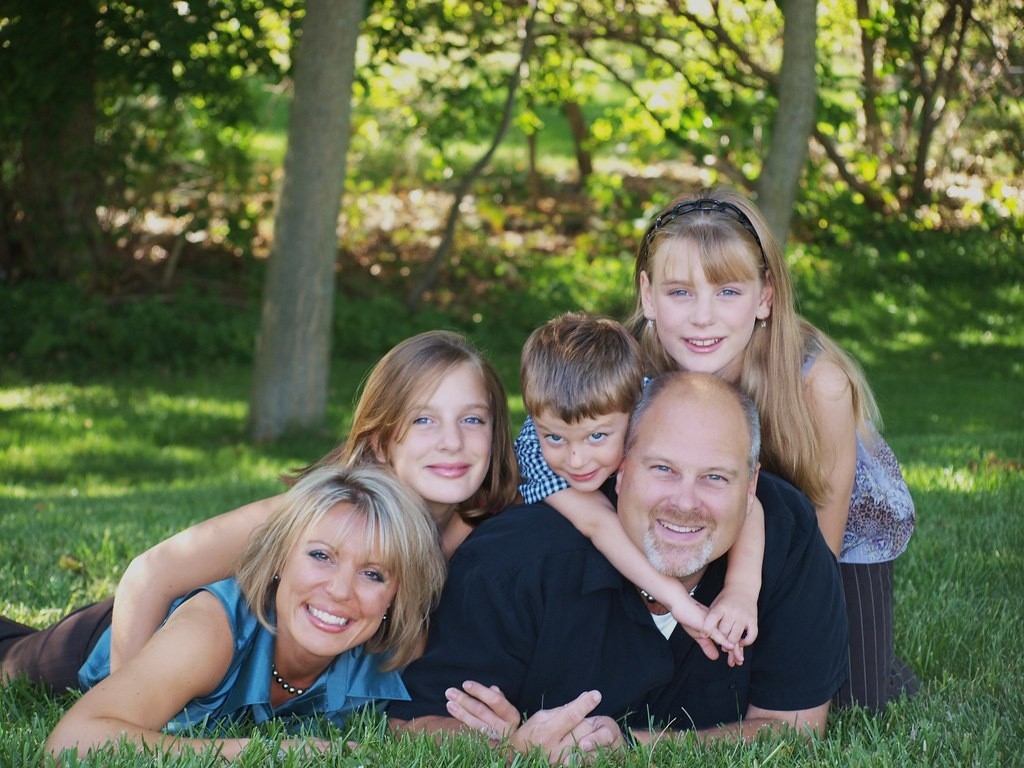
[[389, 372, 847, 767], [623, 191, 921, 719], [512, 312, 766, 667], [0, 466, 520, 768], [110, 330, 517, 676]]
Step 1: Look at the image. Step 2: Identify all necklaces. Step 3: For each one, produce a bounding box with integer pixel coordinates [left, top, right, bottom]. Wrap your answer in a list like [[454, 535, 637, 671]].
[[640, 585, 697, 602], [272, 662, 304, 695]]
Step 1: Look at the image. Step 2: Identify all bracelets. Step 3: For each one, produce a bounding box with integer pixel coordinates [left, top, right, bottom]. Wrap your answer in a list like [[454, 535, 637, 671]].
[[622, 729, 641, 761], [264, 738, 286, 760]]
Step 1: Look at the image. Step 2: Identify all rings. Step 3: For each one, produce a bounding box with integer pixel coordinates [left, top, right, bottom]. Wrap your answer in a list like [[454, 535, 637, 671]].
[[479, 726, 498, 740]]
